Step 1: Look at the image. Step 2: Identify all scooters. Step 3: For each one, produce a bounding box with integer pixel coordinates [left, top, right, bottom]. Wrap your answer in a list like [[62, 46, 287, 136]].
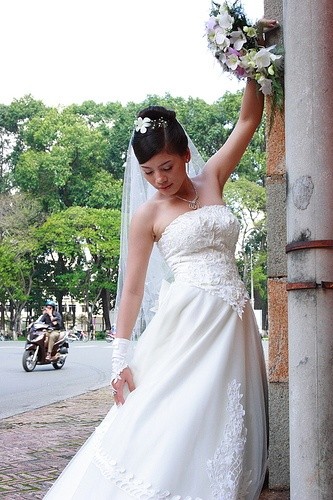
[[0, 328, 23, 342], [104, 329, 115, 343]]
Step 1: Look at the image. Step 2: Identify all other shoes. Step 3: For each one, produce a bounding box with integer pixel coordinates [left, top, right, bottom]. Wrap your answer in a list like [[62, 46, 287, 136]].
[[45, 355, 51, 360]]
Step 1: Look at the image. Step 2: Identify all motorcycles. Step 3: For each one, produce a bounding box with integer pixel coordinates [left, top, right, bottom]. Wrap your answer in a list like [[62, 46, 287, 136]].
[[22, 316, 73, 372], [66, 328, 89, 343]]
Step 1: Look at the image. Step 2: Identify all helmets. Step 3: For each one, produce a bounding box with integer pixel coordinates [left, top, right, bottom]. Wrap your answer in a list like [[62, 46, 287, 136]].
[[46, 301, 55, 309]]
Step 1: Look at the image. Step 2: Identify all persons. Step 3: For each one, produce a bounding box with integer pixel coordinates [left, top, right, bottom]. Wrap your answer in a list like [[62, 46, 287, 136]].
[[36, 302, 63, 360], [40, 17, 280, 500]]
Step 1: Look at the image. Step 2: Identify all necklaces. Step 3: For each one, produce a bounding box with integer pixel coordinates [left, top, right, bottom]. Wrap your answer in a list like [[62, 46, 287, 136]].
[[171, 180, 200, 209]]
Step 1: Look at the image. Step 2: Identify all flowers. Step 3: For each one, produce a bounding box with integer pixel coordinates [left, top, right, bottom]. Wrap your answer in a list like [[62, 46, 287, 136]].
[[134, 117, 152, 134], [204, 0, 284, 136]]
